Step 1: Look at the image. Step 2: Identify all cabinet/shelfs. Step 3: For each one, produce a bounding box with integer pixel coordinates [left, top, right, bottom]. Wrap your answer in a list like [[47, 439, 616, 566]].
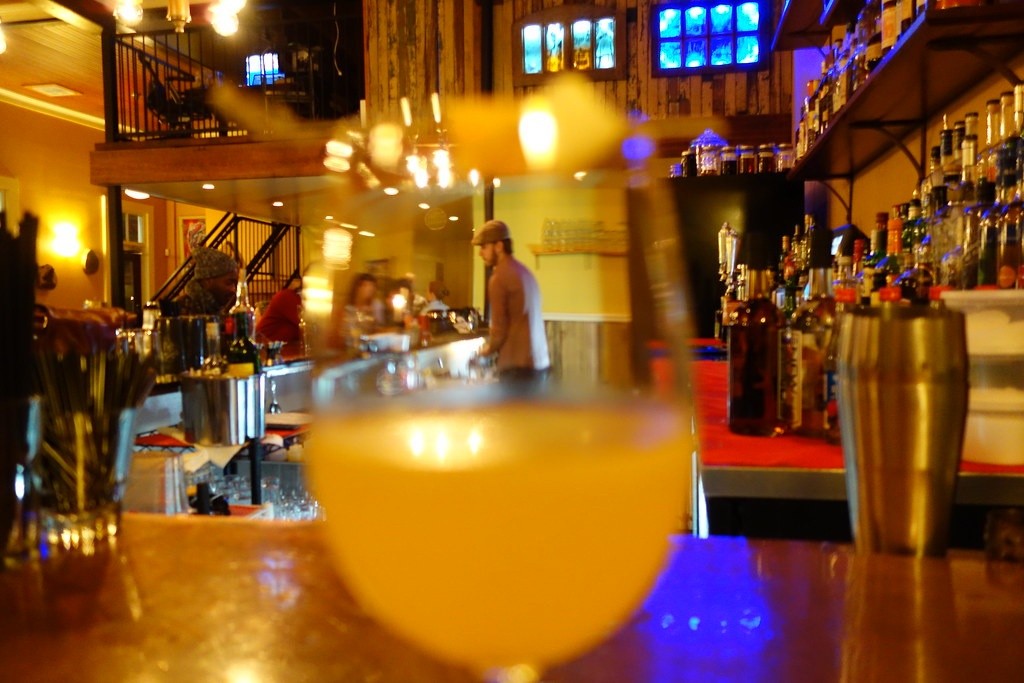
[[767, 0, 1024, 225]]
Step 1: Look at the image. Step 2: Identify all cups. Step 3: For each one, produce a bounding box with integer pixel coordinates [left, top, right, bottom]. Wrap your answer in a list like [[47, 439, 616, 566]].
[[291, 102, 692, 671], [834, 307, 971, 553], [22, 504, 125, 636]]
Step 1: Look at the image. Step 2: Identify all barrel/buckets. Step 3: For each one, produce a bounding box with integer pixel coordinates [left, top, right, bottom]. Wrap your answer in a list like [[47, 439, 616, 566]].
[[181, 372, 269, 445]]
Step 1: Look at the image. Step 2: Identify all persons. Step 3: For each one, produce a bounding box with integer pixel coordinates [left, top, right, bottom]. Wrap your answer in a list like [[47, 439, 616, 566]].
[[419, 281, 451, 317], [167, 248, 235, 314], [343, 273, 384, 348], [471, 221, 551, 383], [256, 288, 302, 345]]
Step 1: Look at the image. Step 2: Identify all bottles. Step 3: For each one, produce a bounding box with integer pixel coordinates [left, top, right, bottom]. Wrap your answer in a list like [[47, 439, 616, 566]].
[[726, 82, 1024, 440], [115, 268, 262, 373], [795, 0, 932, 162]]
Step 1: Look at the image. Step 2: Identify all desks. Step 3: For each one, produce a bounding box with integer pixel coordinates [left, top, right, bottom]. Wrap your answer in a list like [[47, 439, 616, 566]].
[[0, 511, 1024, 682]]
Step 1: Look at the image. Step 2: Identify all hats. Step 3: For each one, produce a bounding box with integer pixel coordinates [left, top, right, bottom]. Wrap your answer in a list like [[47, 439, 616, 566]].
[[471, 220, 510, 246], [192, 246, 237, 282]]
